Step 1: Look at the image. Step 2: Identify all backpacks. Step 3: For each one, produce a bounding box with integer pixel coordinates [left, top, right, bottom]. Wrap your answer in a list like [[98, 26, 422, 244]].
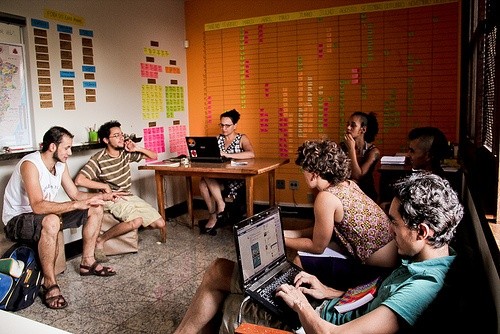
[[0, 246, 43, 311]]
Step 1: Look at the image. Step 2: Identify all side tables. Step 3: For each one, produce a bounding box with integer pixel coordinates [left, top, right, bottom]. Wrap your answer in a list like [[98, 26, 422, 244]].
[[378, 155, 464, 201]]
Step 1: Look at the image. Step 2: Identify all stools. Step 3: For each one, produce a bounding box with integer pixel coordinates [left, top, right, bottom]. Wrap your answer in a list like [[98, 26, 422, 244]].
[[12, 230, 66, 276], [99, 212, 139, 255]]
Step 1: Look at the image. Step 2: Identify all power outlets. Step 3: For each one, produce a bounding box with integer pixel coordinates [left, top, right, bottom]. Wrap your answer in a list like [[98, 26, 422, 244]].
[[276, 180, 285, 189], [289, 181, 299, 189]]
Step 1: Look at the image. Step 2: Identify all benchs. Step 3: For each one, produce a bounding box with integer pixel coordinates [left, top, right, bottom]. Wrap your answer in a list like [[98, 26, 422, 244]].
[[233, 199, 497, 334]]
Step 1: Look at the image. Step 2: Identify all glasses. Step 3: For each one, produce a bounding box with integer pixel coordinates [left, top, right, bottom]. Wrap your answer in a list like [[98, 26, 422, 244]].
[[108, 133, 126, 138], [219, 123, 233, 128]]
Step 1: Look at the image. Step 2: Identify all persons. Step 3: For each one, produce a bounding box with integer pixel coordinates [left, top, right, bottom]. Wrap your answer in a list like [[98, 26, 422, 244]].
[[2, 127, 118, 309], [74, 121, 166, 262], [338, 111, 382, 203], [280, 138, 398, 290], [172, 169, 464, 334], [378, 128, 454, 218], [199, 109, 255, 234]]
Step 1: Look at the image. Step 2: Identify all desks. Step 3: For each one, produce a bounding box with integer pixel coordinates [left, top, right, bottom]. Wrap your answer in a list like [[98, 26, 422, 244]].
[[138, 156, 290, 243]]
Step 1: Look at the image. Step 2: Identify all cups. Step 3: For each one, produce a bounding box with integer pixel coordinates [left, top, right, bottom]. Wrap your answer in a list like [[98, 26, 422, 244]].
[[90, 132, 98, 142]]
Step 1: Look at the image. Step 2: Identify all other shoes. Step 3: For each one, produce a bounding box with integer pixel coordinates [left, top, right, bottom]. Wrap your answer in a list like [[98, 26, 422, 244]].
[[94, 249, 109, 263], [203, 202, 229, 233]]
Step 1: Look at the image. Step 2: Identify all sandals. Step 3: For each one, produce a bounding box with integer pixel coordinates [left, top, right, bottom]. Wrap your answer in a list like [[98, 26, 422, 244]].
[[79, 261, 116, 276], [41, 284, 68, 309]]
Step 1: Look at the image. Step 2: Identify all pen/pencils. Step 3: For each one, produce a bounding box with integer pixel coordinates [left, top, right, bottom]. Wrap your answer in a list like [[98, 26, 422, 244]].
[[11, 148, 24, 151], [89, 123, 96, 131]]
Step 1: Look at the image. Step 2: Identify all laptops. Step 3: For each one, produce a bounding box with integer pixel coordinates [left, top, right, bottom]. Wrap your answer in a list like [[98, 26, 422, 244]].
[[185, 135, 232, 162], [233, 205, 329, 327]]
